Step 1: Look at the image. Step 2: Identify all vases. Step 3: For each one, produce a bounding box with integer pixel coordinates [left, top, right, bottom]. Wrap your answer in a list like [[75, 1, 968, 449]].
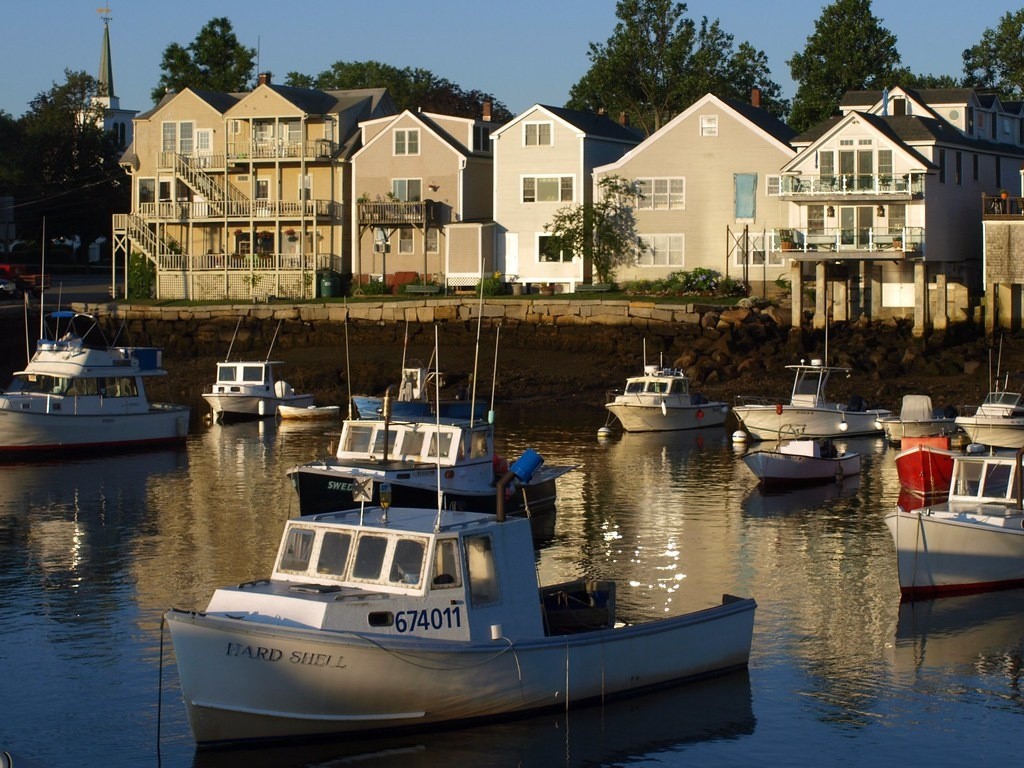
[[511, 282, 522, 296]]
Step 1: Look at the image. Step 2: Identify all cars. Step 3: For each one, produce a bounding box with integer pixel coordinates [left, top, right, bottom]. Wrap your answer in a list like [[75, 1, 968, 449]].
[[0, 278, 16, 300]]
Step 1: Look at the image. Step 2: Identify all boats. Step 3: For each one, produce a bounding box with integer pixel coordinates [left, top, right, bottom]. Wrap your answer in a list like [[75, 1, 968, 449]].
[[288, 258, 581, 518], [954, 333, 1024, 449], [0, 215, 192, 461], [604, 338, 728, 432], [165, 324, 759, 746], [279, 404, 340, 420], [884, 349, 1024, 596], [202, 315, 315, 423], [732, 290, 893, 440], [349, 309, 489, 423], [739, 423, 862, 492], [876, 394, 965, 445]]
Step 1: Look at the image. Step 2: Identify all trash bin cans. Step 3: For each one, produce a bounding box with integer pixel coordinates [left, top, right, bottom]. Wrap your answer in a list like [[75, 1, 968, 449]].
[[315, 267, 334, 299]]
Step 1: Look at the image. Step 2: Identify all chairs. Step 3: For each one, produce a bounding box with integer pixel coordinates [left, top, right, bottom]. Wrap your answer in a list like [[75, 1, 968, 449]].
[[825, 177, 837, 193], [796, 178, 810, 195]]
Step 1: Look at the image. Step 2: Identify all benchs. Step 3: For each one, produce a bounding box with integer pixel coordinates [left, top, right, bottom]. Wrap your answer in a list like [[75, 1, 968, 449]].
[[575, 283, 611, 296], [810, 242, 836, 252], [874, 242, 892, 252], [20, 274, 51, 290], [403, 285, 440, 299]]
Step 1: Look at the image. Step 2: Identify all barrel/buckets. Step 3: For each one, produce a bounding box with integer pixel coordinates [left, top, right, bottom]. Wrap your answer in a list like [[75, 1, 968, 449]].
[[510, 448, 545, 484]]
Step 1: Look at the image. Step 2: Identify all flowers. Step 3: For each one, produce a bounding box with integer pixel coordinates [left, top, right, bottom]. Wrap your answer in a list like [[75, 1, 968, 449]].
[[234, 229, 243, 241], [285, 229, 295, 236], [257, 230, 271, 240]]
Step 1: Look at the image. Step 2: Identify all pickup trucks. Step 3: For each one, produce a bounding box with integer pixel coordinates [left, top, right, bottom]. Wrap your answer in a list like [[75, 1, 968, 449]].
[[0, 264, 51, 299]]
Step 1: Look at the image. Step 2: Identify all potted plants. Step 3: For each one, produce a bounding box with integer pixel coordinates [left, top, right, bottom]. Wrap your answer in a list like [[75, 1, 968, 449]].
[[781, 237, 795, 252], [258, 251, 270, 259], [893, 236, 902, 248], [540, 283, 555, 295], [231, 253, 244, 259], [1000, 189, 1011, 200]]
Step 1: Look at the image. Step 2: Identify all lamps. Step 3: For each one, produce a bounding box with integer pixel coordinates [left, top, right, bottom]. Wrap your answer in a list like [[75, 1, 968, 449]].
[[428, 184, 435, 193], [827, 206, 834, 217], [877, 205, 885, 217]]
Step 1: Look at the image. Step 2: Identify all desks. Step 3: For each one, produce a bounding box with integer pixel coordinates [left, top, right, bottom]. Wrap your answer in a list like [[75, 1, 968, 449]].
[[879, 184, 890, 191]]
